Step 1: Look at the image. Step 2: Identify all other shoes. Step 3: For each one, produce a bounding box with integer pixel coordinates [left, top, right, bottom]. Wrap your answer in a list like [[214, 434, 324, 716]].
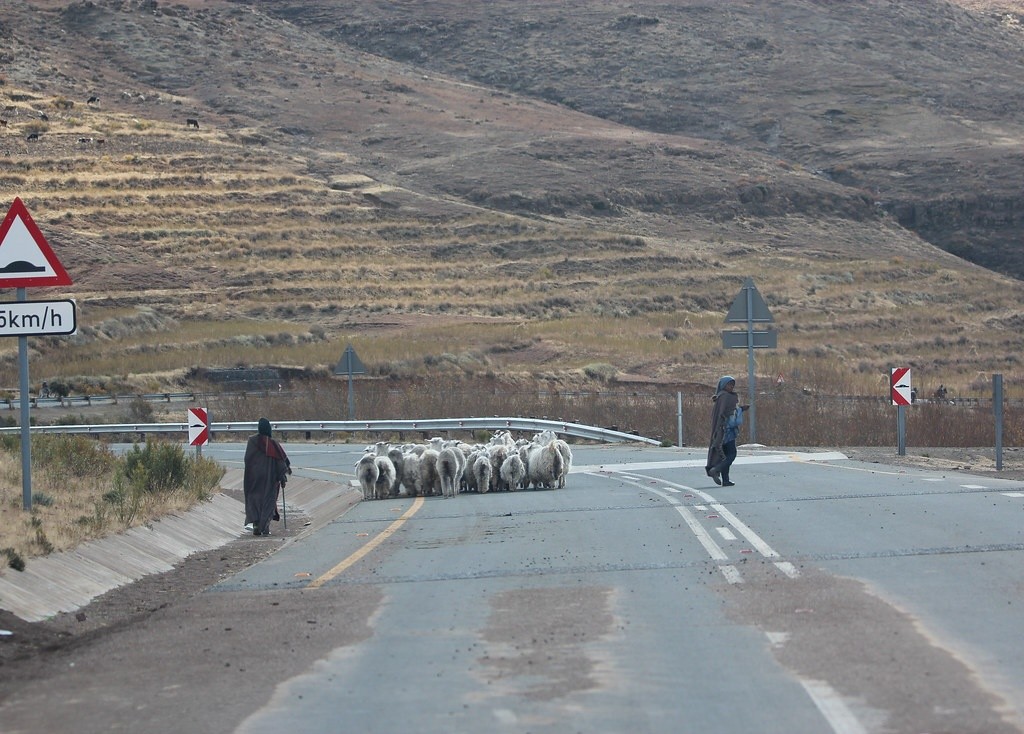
[[253, 529, 261, 534], [722, 481, 735, 486], [708, 467, 721, 485], [261, 531, 271, 535]]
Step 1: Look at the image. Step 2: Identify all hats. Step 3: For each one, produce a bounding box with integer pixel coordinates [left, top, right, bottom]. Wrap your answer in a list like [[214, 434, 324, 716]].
[[258, 419, 270, 436], [719, 376, 735, 389]]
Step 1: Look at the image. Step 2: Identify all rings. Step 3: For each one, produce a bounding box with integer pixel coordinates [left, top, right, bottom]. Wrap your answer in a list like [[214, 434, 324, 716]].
[[285, 484, 286, 485]]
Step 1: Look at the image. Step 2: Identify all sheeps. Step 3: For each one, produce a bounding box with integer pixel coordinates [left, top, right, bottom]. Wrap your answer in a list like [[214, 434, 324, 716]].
[[354, 430, 573, 501]]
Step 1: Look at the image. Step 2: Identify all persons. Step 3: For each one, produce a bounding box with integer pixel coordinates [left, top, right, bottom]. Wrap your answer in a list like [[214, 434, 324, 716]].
[[936, 384, 943, 398], [710, 376, 750, 487], [244, 418, 285, 536]]
